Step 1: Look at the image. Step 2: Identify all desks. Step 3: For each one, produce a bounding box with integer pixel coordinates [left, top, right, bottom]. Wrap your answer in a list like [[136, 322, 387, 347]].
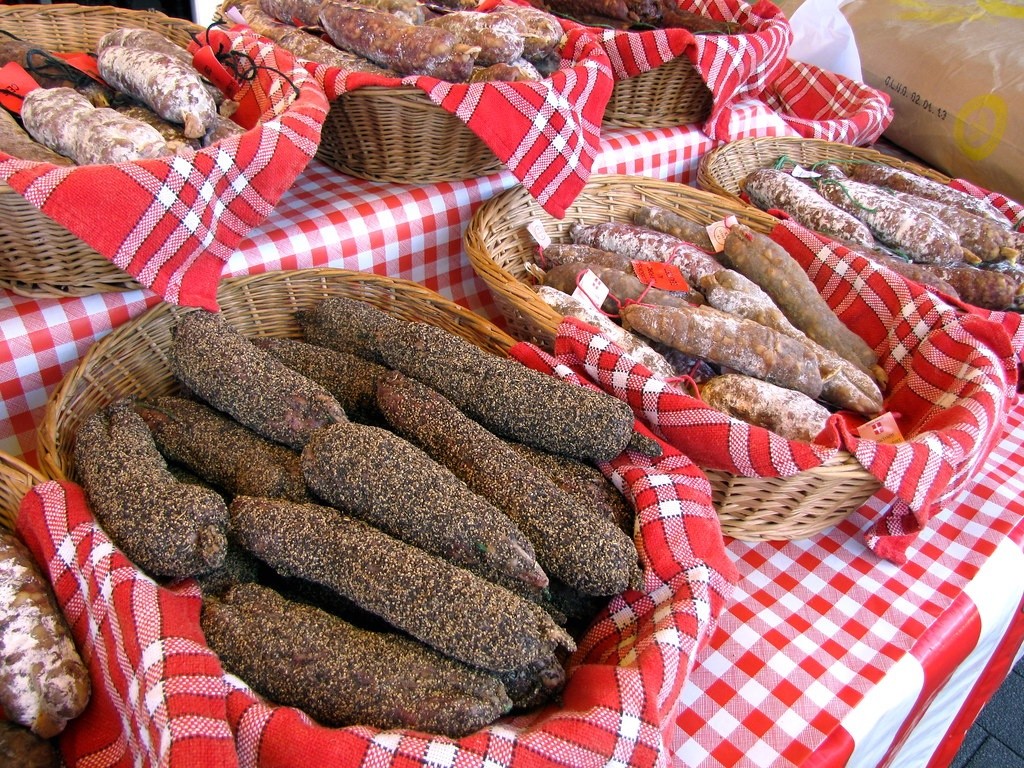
[[0, 0, 1024, 768]]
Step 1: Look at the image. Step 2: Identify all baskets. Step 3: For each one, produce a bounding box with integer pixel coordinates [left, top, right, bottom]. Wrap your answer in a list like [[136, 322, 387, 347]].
[[0, 0, 1024, 720]]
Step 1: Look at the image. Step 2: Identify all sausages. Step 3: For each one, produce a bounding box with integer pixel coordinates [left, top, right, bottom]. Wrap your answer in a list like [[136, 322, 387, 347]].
[[0, 0, 1024, 768]]
